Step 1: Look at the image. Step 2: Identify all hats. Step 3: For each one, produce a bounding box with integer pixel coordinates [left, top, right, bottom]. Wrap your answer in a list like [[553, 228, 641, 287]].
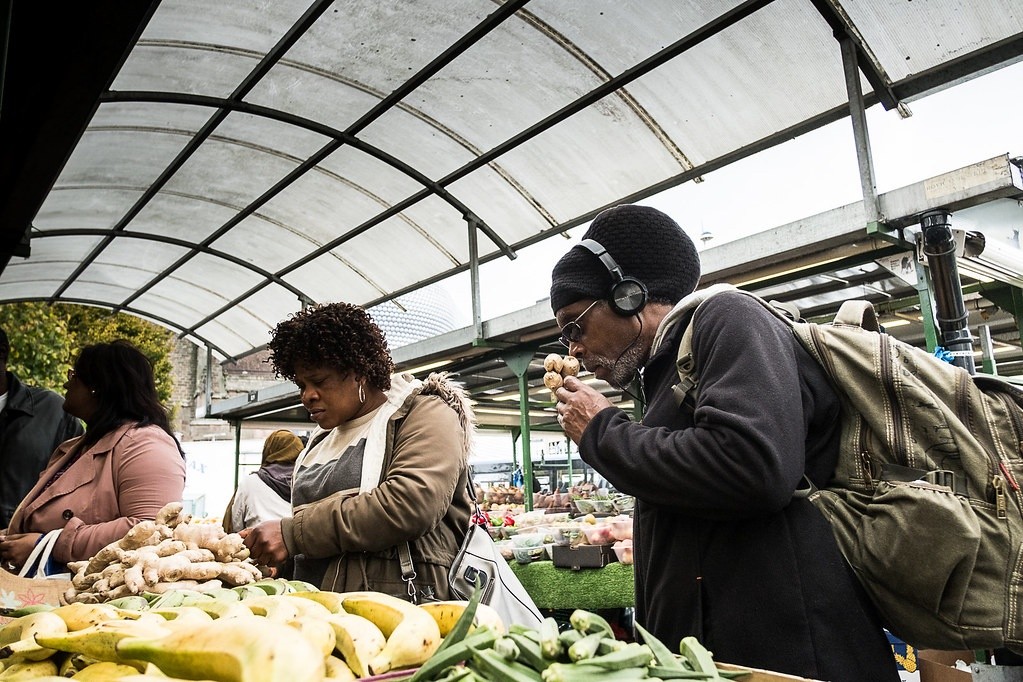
[[551, 203, 700, 315]]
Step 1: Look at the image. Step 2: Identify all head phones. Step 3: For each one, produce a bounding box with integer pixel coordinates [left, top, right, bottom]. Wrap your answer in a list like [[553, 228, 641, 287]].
[[572, 239, 648, 318]]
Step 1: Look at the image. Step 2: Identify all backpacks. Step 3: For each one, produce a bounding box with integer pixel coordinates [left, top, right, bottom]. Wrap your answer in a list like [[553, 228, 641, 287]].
[[670, 288, 1023, 650]]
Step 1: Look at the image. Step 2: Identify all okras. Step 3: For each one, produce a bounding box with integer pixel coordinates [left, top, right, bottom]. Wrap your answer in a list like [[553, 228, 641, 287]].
[[398, 571, 754, 682]]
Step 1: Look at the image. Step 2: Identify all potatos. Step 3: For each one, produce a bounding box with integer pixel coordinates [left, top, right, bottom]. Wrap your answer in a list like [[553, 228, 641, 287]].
[[529, 485, 585, 508]]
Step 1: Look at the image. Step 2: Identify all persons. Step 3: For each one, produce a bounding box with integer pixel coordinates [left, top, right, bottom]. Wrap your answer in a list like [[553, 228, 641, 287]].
[[222, 429, 305, 536], [551, 204, 901, 682], [239, 301, 472, 606], [0, 327, 85, 536], [0, 339, 186, 579], [517, 463, 540, 493]]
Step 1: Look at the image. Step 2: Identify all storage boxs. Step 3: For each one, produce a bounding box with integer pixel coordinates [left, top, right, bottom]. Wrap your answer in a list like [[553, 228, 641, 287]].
[[486, 498, 634, 570], [885, 631, 1023, 682]]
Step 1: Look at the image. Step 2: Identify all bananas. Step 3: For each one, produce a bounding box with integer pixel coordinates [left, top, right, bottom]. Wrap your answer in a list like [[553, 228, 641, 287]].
[[0, 577, 505, 682]]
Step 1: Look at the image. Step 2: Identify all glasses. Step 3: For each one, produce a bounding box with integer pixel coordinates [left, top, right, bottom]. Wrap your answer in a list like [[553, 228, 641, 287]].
[[557, 300, 600, 350], [67, 369, 78, 382]]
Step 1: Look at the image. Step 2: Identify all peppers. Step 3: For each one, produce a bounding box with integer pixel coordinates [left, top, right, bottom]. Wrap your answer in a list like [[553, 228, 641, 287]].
[[472, 511, 518, 540]]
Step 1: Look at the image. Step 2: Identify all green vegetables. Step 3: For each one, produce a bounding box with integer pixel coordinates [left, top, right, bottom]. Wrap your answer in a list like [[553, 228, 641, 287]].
[[571, 493, 636, 515]]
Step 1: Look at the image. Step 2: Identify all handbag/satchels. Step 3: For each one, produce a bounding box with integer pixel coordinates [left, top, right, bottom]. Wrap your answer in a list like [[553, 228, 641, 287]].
[[0, 528, 73, 608], [448, 512, 544, 629]]
